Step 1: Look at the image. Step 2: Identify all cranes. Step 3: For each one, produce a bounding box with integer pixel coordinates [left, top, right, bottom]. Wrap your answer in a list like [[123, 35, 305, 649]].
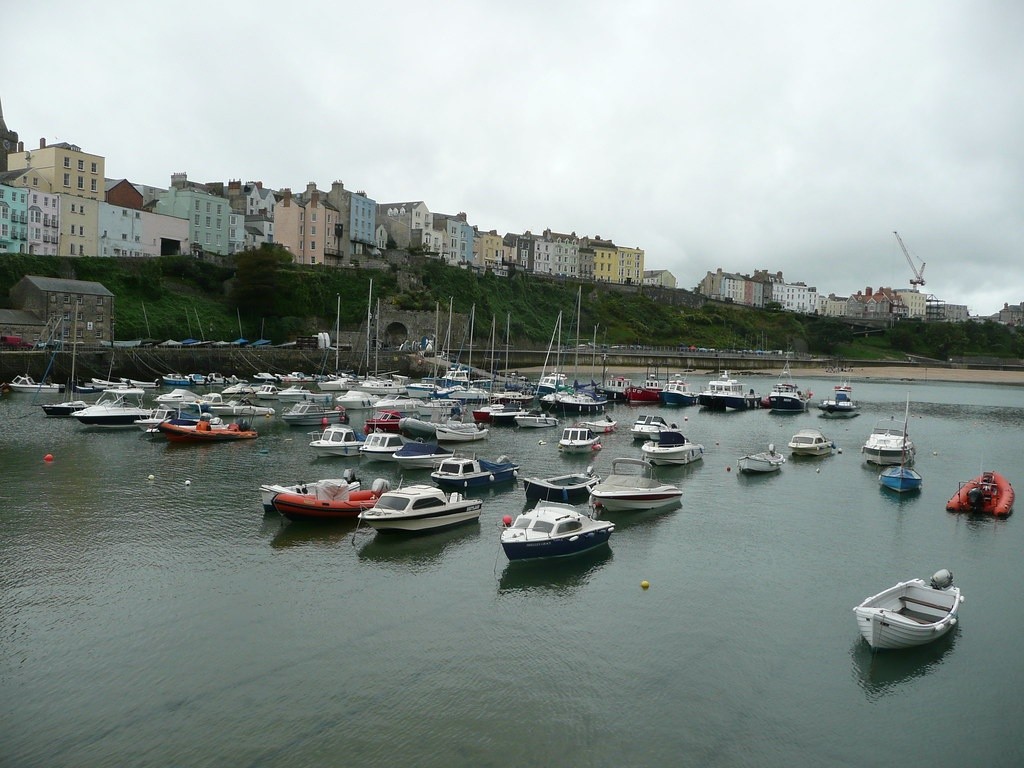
[[892, 230, 926, 290]]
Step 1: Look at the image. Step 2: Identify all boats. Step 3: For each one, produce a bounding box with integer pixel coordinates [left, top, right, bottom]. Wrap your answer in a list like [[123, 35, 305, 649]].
[[392, 441, 464, 471], [99, 339, 141, 348], [280, 395, 349, 425], [307, 424, 366, 458], [523, 465, 601, 504], [698, 359, 762, 413], [738, 444, 786, 473], [69, 352, 175, 429], [431, 449, 521, 488], [656, 374, 700, 406], [852, 568, 965, 652], [577, 415, 617, 433], [557, 427, 603, 456], [134, 402, 230, 439], [358, 433, 416, 463], [818, 373, 858, 419], [626, 373, 664, 405], [787, 428, 836, 457], [53, 339, 86, 347], [640, 430, 705, 468], [356, 485, 483, 537], [768, 359, 813, 412], [157, 401, 258, 442], [945, 470, 1015, 519], [258, 469, 389, 524], [7, 373, 66, 393], [861, 416, 915, 466], [500, 497, 616, 563], [631, 415, 669, 441], [586, 458, 683, 513]]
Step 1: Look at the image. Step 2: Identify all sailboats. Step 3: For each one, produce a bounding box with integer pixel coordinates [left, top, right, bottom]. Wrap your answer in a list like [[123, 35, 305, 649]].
[[30, 299, 91, 418], [880, 391, 922, 494], [74, 277, 633, 444]]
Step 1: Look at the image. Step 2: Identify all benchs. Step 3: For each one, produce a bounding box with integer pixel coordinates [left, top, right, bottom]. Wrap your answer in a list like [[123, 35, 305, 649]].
[[899, 596, 951, 612]]
